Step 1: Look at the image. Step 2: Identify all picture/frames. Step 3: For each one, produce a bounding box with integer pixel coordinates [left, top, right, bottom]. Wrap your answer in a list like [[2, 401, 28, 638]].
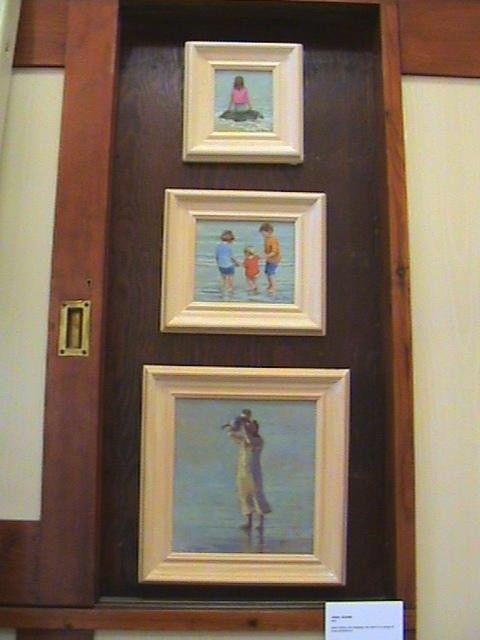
[[137, 363, 348, 587], [180, 41, 306, 165], [161, 185, 329, 337]]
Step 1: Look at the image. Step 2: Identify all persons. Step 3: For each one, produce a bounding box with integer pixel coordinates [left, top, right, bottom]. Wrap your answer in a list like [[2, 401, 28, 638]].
[[260, 224, 280, 290], [242, 248, 261, 290], [229, 419, 272, 530], [212, 231, 238, 289], [219, 409, 252, 431], [225, 77, 252, 113]]
